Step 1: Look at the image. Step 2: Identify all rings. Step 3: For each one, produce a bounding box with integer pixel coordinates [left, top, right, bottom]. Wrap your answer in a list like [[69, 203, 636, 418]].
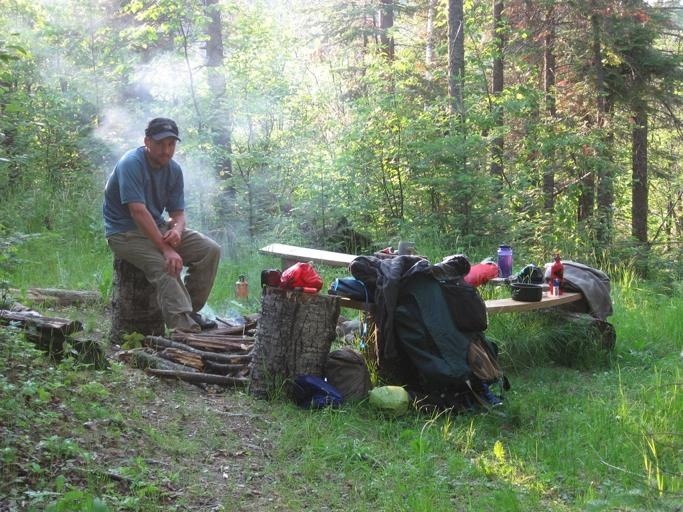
[[174, 241, 176, 244]]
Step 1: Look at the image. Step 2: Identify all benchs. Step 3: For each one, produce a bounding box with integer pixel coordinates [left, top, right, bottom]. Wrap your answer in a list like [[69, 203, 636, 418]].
[[485, 290, 583, 315], [257, 243, 551, 289]]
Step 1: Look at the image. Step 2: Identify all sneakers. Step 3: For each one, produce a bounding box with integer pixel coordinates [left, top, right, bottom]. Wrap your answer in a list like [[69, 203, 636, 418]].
[[191, 313, 218, 332]]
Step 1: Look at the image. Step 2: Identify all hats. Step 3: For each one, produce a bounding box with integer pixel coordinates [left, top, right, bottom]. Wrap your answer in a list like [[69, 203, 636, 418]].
[[145, 118, 182, 143]]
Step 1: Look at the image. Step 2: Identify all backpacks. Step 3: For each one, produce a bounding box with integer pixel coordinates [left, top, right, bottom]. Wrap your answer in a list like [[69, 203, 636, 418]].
[[350, 255, 508, 406]]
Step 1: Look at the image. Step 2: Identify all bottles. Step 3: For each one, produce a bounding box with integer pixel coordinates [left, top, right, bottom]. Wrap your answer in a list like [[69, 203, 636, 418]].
[[235, 275, 248, 299], [551, 254, 564, 295]]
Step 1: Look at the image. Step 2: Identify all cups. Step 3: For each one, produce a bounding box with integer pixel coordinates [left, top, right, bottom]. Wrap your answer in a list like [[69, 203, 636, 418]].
[[498, 244, 512, 277]]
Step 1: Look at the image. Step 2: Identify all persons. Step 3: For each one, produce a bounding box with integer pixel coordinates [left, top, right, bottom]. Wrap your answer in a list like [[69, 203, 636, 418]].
[[102, 118, 221, 334]]
